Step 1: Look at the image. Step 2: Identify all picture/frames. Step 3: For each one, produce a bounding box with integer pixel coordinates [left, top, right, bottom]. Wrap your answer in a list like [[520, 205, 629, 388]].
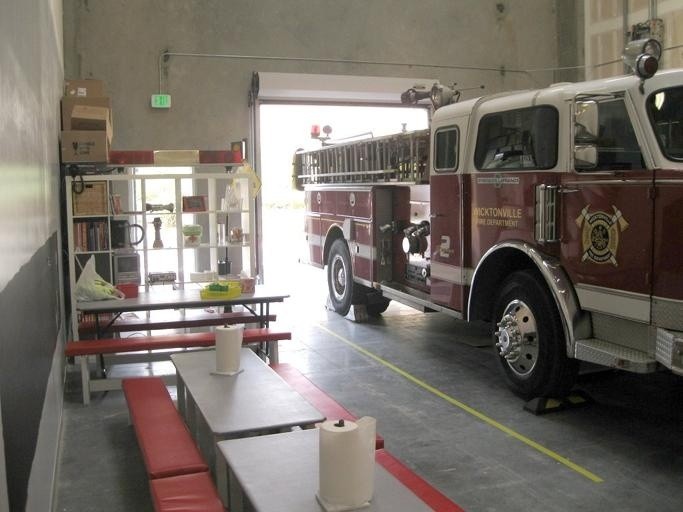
[[181, 195, 206, 212]]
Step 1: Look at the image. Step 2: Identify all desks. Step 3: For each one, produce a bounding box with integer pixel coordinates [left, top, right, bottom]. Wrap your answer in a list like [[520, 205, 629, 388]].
[[214, 427, 435, 512], [76, 283, 290, 393], [171, 346, 326, 434]]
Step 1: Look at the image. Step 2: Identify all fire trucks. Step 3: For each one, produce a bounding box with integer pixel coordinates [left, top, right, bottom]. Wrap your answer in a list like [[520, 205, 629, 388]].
[[291, 38, 683, 403]]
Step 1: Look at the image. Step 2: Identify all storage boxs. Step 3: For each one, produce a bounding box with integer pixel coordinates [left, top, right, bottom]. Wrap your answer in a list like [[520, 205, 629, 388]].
[[58, 79, 113, 164]]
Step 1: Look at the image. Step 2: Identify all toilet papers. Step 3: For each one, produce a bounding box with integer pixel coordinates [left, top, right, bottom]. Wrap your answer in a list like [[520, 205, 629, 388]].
[[215, 323, 246, 375], [317, 416, 377, 507]]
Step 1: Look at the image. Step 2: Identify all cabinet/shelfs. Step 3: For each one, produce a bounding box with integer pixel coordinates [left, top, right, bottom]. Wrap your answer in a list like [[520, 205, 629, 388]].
[[64, 172, 257, 342]]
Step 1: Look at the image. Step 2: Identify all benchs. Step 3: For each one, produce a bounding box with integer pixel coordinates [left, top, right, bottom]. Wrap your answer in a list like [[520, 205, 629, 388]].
[[374, 448, 464, 511], [63, 311, 291, 404], [121, 375, 228, 512]]
